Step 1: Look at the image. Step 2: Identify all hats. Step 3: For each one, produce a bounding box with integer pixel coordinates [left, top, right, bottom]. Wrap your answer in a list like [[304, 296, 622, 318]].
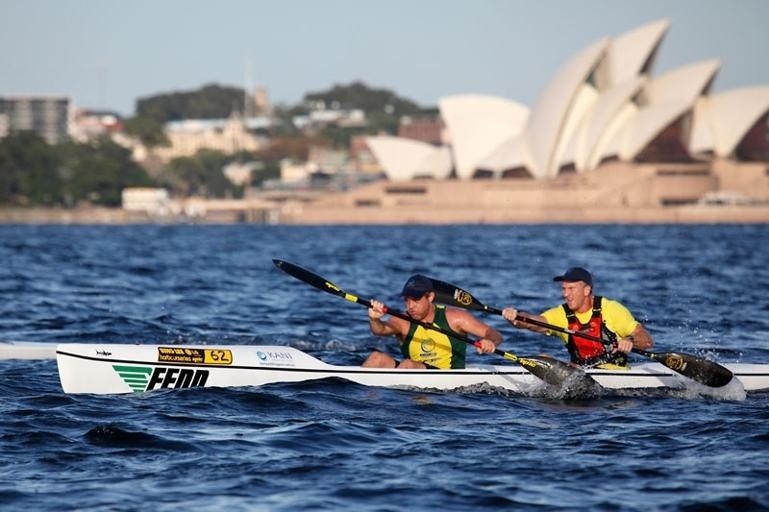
[[553, 267, 593, 288], [399, 274, 433, 298]]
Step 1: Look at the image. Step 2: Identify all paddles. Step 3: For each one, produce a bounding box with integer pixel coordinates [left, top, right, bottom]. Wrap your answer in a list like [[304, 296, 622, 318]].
[[426, 276, 732, 387], [272, 259, 605, 397]]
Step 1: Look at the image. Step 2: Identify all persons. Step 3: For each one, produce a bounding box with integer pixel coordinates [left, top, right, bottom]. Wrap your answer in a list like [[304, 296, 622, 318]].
[[349, 272, 504, 371], [500, 265, 657, 369]]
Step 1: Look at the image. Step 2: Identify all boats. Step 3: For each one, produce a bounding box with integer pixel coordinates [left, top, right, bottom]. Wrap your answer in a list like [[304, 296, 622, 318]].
[[52, 342, 769, 400]]
